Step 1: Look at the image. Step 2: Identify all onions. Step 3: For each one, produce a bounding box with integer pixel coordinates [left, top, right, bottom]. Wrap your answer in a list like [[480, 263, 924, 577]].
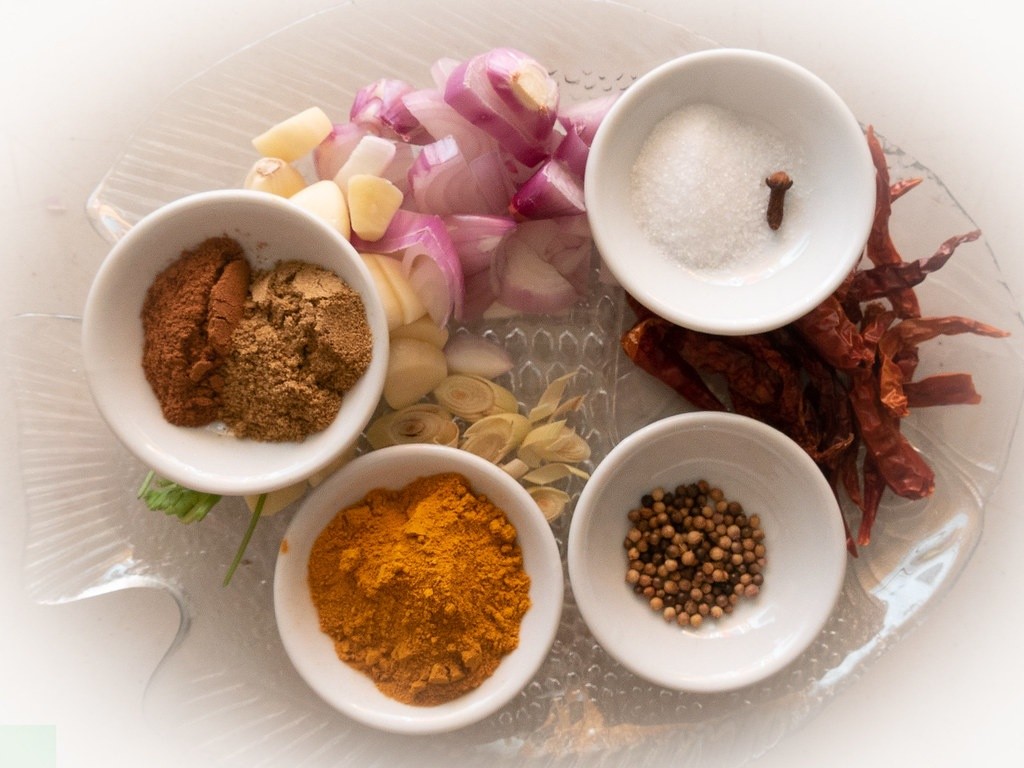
[[309, 49, 619, 380]]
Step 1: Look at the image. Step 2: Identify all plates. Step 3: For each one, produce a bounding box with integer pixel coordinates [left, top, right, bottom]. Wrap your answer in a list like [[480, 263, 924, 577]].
[[567, 410, 849, 694], [272, 442, 565, 738], [584, 51, 877, 335], [78, 191, 389, 495], [0, 0, 1024, 768]]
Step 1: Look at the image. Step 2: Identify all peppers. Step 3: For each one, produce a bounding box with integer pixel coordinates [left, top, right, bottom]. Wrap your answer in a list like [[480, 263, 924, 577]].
[[621, 125, 1012, 555]]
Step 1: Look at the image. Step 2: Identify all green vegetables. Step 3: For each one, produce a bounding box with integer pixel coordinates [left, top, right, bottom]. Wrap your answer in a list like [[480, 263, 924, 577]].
[[135, 472, 267, 589]]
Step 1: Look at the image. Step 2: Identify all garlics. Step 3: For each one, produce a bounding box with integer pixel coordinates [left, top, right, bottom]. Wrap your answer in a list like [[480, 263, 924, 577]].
[[240, 106, 448, 517]]
